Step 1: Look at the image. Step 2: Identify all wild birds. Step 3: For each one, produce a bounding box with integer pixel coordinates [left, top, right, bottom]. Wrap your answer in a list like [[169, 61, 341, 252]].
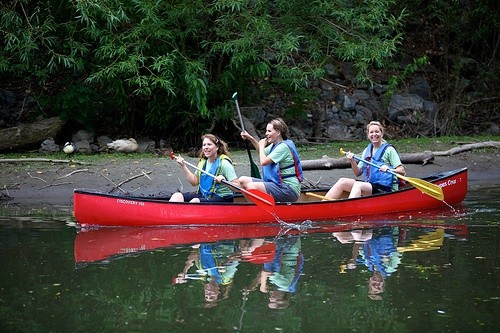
[[63, 141, 76, 154], [106, 138, 139, 156], [88, 142, 108, 155]]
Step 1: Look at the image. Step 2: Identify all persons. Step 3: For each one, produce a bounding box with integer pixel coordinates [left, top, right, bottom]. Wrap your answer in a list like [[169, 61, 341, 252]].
[[238, 118, 305, 201], [179, 242, 240, 309], [335, 230, 403, 302], [242, 236, 307, 308], [321, 121, 406, 201], [167, 134, 241, 203]]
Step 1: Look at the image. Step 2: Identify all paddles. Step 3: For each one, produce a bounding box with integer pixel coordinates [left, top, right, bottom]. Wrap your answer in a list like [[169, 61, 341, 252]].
[[232, 92, 262, 180], [172, 241, 276, 285], [339, 229, 444, 274], [170, 151, 276, 214], [340, 147, 444, 201]]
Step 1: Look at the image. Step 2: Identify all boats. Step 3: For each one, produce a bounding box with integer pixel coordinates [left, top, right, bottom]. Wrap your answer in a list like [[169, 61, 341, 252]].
[[71, 166, 469, 227], [73, 203, 471, 270]]
